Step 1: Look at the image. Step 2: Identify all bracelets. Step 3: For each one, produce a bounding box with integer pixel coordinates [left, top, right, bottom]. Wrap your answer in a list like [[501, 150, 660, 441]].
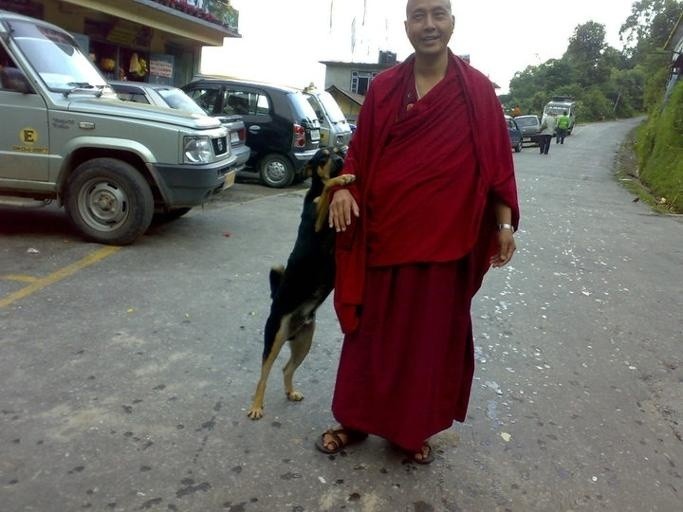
[[496, 224, 515, 234]]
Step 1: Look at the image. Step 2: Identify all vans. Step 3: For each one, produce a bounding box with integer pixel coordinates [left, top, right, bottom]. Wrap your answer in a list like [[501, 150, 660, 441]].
[[541, 96, 576, 135], [502, 114, 524, 152]]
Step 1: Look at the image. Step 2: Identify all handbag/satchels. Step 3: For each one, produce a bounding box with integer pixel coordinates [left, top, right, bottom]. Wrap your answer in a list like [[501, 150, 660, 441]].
[[542, 121, 547, 129]]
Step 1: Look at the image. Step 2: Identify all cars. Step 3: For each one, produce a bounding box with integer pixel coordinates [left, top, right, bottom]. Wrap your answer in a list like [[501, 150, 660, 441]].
[[292, 87, 354, 149], [513, 114, 543, 145], [108, 79, 252, 173]]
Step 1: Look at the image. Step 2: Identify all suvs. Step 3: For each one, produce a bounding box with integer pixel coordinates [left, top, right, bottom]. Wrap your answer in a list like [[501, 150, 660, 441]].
[[179, 78, 321, 188], [0, 9, 239, 246]]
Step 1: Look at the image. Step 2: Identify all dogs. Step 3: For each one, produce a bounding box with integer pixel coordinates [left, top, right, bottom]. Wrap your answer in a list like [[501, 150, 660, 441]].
[[245, 147, 358, 420]]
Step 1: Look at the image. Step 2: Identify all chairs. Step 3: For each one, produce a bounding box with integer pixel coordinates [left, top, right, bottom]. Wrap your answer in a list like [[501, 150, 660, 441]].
[[225, 91, 252, 115]]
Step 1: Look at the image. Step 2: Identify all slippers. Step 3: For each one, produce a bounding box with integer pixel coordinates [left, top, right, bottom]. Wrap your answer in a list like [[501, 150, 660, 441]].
[[413, 441, 434, 464], [314, 424, 367, 452]]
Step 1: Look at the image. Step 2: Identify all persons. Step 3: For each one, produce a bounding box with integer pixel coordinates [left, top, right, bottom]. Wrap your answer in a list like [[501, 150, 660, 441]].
[[556, 112, 570, 144], [538, 111, 555, 155], [314, 1, 520, 465], [507, 104, 523, 125]]
